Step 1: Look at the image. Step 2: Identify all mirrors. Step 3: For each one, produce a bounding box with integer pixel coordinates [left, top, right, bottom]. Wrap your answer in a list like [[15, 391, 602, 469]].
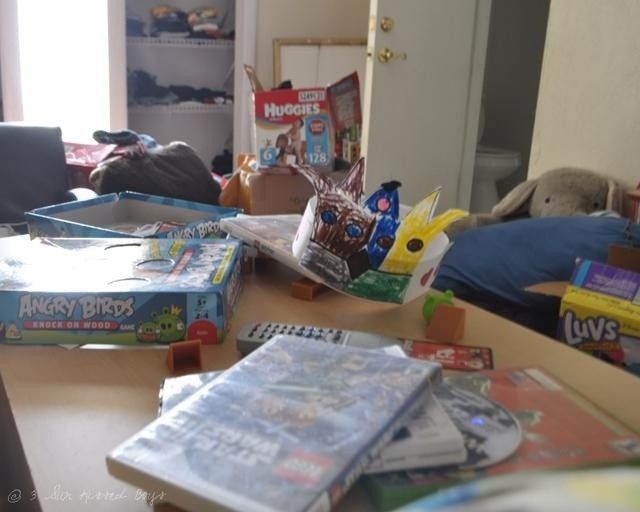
[[273, 38, 369, 85]]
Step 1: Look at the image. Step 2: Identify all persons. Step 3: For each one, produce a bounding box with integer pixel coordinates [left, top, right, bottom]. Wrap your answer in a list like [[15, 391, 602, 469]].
[[286, 118, 303, 164], [431, 216, 640, 338]]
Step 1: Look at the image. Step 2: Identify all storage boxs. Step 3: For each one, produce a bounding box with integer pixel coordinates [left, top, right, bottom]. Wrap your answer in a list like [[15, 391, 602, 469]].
[[237, 166, 351, 221], [519, 246, 639, 374], [0, 193, 251, 361]]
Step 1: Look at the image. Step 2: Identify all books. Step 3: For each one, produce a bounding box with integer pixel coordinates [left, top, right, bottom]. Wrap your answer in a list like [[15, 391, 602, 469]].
[[220, 214, 323, 282], [107, 333, 640, 512]]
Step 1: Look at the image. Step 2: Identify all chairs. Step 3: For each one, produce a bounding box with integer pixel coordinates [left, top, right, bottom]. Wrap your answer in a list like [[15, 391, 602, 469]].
[[1, 123, 97, 233]]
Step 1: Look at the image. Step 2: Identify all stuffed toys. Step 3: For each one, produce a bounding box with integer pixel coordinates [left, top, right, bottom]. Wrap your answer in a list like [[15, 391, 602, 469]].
[[443, 168, 623, 239]]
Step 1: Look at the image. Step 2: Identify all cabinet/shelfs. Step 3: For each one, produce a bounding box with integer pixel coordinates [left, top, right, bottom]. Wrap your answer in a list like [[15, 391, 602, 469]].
[[109, 1, 254, 116]]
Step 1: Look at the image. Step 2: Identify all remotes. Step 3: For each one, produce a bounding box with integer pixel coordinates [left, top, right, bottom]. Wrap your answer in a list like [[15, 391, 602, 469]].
[[236, 321, 405, 356]]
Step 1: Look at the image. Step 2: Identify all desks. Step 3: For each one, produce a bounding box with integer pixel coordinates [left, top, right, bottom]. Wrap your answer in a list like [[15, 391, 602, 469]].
[[2, 260, 638, 510]]
